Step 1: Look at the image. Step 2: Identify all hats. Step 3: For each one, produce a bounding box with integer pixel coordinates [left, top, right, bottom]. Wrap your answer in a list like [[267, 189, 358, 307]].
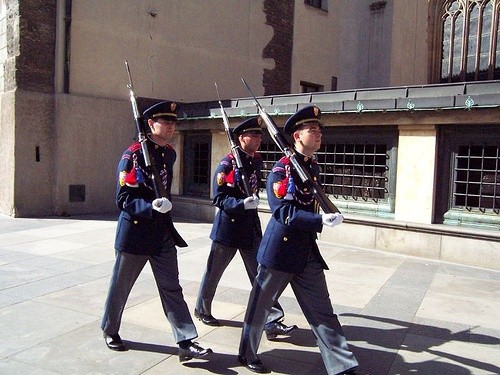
[[283, 106, 324, 136], [231, 117, 266, 136], [142, 100, 181, 122]]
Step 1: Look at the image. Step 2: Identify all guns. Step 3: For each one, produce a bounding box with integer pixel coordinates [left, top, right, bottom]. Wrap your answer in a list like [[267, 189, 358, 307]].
[[240, 77, 344, 223], [213, 81, 260, 202], [124, 59, 170, 207]]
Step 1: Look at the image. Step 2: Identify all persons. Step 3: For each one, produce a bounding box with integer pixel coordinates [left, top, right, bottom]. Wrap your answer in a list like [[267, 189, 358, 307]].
[[194, 117, 299, 339], [238, 106, 359, 375], [100, 101, 213, 362]]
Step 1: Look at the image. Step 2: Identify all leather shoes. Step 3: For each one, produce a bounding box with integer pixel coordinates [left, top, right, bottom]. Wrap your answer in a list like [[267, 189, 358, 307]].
[[340, 370, 372, 375], [103, 330, 125, 350], [195, 308, 218, 324], [265, 319, 299, 341], [238, 354, 267, 372], [179, 340, 213, 364]]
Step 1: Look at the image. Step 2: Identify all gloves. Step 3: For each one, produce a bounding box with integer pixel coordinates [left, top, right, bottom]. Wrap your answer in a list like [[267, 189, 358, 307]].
[[322, 211, 344, 227], [152, 196, 173, 214], [244, 193, 259, 209]]
